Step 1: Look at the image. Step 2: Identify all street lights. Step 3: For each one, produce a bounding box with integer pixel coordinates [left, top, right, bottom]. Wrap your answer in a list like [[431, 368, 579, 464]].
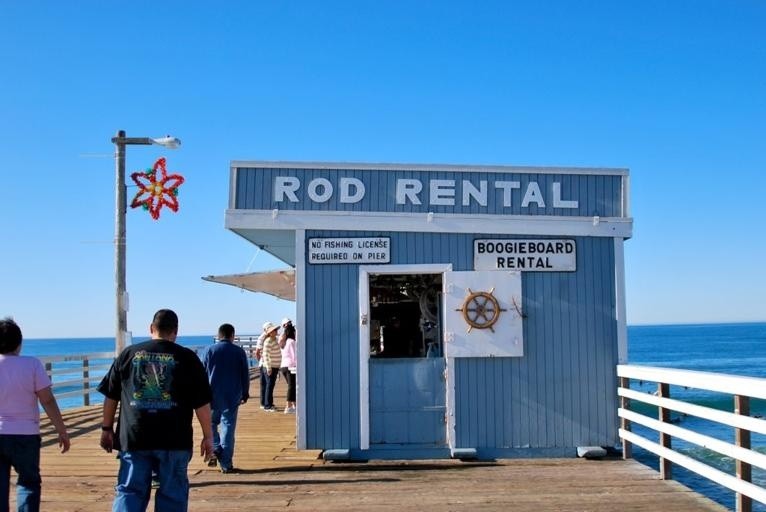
[[111, 130, 181, 362]]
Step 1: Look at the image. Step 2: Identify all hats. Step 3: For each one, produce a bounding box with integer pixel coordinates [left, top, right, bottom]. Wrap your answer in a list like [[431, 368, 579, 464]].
[[281, 317, 291, 327], [262, 322, 272, 328], [263, 325, 280, 340]]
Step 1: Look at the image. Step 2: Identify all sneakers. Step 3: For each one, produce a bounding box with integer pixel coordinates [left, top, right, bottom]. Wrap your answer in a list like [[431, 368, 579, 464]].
[[260, 404, 296, 414], [220, 467, 233, 473], [207, 453, 217, 467]]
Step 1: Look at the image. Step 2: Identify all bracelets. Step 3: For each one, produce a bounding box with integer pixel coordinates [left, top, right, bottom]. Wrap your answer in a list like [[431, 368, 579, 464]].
[[102, 426, 113, 432]]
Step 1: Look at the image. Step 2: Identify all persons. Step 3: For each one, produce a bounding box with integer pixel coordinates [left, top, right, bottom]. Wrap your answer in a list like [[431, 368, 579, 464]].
[[256, 318, 296, 414], [96, 309, 213, 512], [0, 319, 70, 512], [200, 324, 249, 473]]
